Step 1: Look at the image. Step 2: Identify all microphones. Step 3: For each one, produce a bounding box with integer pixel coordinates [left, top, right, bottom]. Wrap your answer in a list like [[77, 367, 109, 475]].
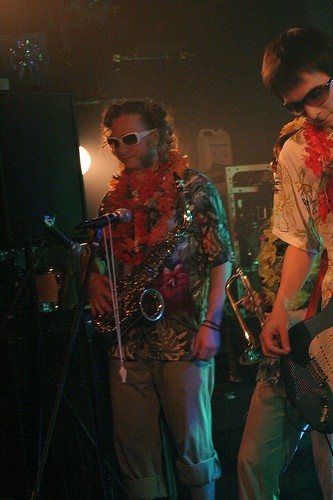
[[74, 208, 132, 230], [41, 216, 80, 253]]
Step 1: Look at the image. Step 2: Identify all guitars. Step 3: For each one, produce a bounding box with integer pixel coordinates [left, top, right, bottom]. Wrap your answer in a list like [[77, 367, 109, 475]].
[[279, 296, 333, 435]]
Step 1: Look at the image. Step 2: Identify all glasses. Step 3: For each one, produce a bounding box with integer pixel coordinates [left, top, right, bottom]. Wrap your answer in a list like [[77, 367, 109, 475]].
[[108, 127, 158, 150], [283, 77, 333, 115]]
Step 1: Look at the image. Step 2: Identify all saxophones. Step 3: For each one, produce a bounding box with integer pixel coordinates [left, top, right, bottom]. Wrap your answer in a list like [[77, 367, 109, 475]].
[[91, 172, 192, 345]]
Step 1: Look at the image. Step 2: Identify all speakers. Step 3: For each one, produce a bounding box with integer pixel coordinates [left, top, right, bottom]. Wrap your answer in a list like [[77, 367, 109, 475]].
[[0, 241, 126, 500], [0, 90, 90, 251]]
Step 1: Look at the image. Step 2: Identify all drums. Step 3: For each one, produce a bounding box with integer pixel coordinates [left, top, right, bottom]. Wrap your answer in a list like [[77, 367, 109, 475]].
[[0, 240, 109, 346]]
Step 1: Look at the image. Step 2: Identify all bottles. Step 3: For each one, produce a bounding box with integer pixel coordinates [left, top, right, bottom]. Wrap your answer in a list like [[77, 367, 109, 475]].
[[237, 199, 250, 238]]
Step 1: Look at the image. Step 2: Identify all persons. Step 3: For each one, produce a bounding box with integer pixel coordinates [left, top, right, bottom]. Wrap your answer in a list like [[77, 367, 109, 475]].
[[79, 98, 234, 500], [236, 24, 333, 500]]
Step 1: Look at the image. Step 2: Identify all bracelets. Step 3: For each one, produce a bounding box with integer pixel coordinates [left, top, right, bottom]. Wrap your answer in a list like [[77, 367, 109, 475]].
[[201, 319, 223, 331]]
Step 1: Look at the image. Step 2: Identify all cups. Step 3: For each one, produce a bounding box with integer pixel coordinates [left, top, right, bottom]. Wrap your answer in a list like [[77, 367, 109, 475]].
[[33, 267, 62, 312]]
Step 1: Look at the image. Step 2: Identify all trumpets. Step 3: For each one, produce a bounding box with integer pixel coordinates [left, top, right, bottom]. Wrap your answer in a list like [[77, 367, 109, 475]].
[[226, 252, 283, 395]]
